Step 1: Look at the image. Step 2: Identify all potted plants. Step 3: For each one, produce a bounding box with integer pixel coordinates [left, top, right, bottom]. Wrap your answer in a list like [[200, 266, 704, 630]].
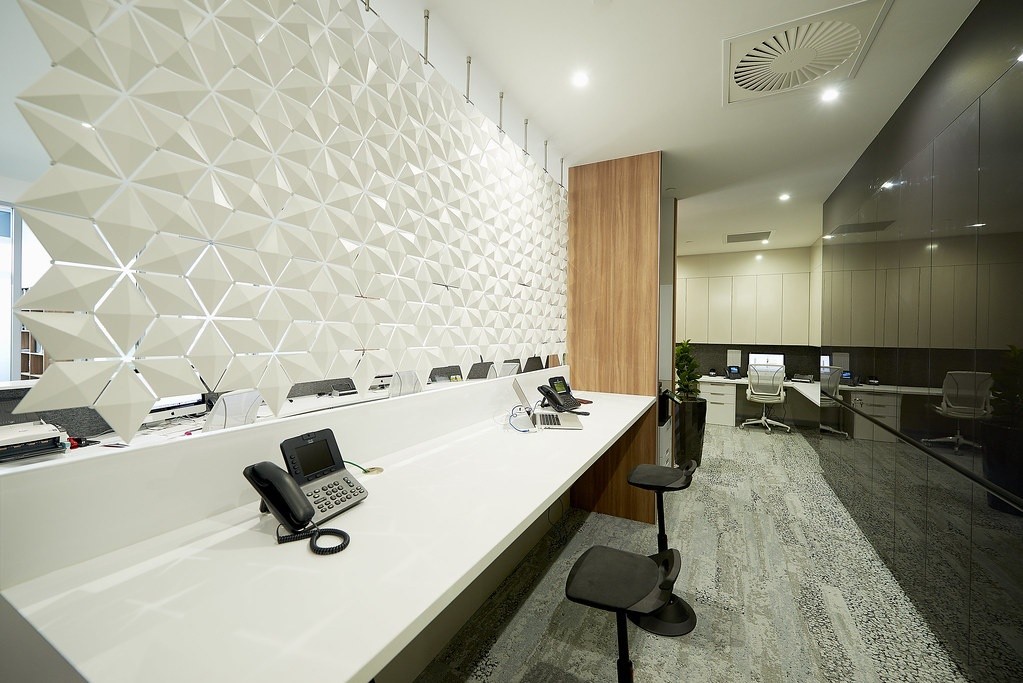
[[978, 344, 1023, 516], [674, 339, 707, 467]]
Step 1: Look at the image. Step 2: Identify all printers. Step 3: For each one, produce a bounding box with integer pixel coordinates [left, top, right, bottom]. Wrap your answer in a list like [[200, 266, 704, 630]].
[[791, 373, 814, 383], [0, 396, 71, 464]]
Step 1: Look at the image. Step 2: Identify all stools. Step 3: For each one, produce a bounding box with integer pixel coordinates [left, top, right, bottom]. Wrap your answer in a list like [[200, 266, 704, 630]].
[[627, 459, 697, 637], [566, 547, 681, 683]]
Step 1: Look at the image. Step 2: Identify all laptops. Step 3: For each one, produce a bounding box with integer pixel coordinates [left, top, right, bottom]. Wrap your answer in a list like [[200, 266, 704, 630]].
[[512, 378, 583, 430]]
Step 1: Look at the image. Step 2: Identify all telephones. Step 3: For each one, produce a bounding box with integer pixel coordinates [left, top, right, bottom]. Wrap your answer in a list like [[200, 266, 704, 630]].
[[537, 376, 581, 413], [725, 366, 741, 379], [244, 429, 367, 536]]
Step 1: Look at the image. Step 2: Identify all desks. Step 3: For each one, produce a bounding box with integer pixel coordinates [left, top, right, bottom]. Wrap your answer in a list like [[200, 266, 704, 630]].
[[696, 375, 820, 426], [1, 366, 657, 683], [839, 384, 1020, 443]]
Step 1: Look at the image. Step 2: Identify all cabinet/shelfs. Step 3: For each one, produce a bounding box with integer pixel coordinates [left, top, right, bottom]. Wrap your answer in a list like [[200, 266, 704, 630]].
[[19, 287, 74, 380]]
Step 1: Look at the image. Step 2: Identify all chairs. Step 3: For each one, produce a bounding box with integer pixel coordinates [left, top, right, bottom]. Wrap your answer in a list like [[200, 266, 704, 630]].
[[739, 363, 791, 434], [820, 366, 848, 441], [921, 371, 994, 454]]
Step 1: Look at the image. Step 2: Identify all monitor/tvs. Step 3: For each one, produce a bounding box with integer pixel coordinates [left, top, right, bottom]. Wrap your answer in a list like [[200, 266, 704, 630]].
[[73, 281, 206, 425], [747, 352, 786, 378], [820, 354, 831, 379]]
